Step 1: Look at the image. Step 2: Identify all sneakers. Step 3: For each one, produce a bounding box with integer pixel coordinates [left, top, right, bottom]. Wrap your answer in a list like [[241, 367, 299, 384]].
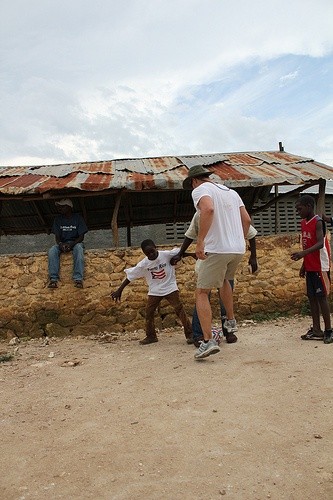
[[323, 328, 333, 344], [194, 339, 220, 360], [221, 316, 239, 334], [301, 327, 323, 340]]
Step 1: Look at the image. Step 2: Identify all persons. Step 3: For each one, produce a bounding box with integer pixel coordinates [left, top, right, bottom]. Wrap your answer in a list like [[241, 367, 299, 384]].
[[183, 165, 251, 358], [47, 198, 85, 288], [170, 175, 258, 348], [110, 239, 198, 344], [291, 195, 333, 343]]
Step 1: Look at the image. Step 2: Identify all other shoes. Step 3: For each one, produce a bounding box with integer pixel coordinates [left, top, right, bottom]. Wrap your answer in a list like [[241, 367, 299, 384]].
[[48, 282, 58, 288], [74, 280, 83, 288], [186, 335, 194, 344], [226, 334, 237, 343], [139, 337, 159, 345], [194, 340, 201, 347]]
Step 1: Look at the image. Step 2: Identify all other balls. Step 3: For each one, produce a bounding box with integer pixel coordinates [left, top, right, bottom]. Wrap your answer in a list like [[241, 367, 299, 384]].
[[211, 327, 224, 344]]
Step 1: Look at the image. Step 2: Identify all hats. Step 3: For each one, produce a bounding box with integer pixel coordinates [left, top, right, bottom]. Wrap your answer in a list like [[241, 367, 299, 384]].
[[55, 199, 74, 209], [182, 165, 216, 190]]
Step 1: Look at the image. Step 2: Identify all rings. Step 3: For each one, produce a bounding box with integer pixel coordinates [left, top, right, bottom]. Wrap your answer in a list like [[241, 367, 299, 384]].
[[68, 248, 70, 250]]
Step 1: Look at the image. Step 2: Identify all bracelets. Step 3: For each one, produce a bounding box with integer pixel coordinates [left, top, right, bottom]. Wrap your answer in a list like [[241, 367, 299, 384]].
[[59, 242, 62, 244]]
